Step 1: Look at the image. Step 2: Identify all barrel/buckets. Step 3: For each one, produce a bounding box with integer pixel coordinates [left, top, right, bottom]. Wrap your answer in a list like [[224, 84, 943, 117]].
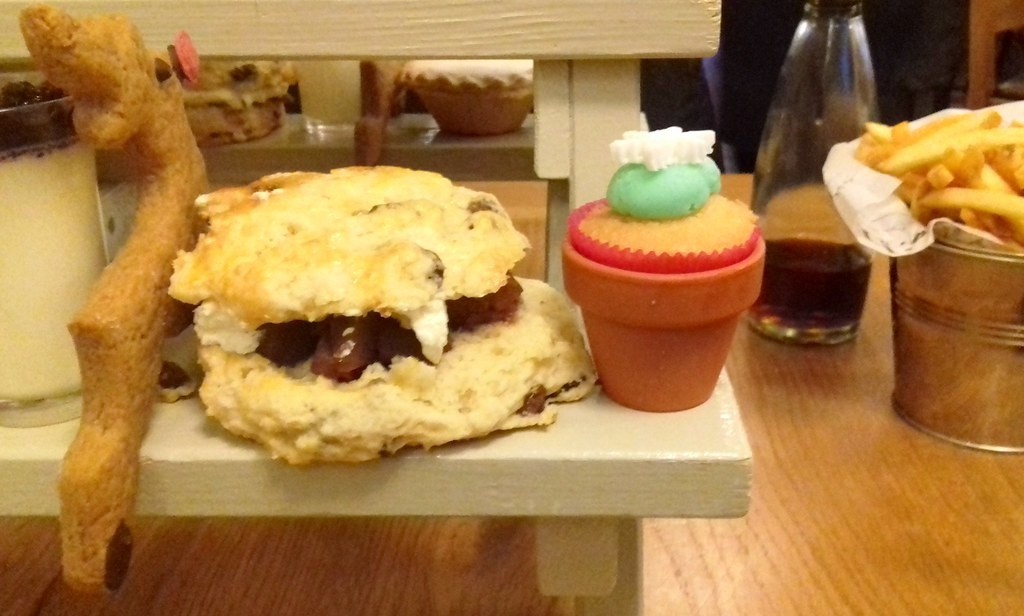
[[885, 241, 1024, 455]]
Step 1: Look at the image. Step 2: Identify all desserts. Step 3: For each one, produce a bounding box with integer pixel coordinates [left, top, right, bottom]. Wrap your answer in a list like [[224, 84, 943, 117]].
[[564, 124, 761, 275]]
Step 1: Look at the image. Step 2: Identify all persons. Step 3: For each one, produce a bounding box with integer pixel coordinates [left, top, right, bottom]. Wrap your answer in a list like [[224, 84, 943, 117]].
[[702, 0, 918, 176]]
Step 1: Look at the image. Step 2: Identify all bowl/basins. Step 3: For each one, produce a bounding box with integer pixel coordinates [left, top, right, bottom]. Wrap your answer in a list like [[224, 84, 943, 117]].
[[399, 69, 533, 137], [560, 236, 772, 414]]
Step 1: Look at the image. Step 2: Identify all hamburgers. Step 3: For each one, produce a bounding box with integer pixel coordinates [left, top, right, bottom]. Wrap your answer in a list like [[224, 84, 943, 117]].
[[172, 164, 598, 463]]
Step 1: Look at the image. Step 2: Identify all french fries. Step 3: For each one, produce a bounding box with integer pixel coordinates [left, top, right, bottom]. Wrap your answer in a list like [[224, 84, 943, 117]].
[[857, 109, 1024, 249]]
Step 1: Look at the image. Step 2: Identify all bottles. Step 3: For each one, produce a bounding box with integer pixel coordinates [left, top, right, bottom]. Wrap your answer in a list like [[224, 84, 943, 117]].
[[749, 0, 880, 347]]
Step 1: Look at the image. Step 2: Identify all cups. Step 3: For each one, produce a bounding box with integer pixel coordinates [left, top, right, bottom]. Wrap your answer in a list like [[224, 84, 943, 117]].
[[0, 97, 110, 433]]
